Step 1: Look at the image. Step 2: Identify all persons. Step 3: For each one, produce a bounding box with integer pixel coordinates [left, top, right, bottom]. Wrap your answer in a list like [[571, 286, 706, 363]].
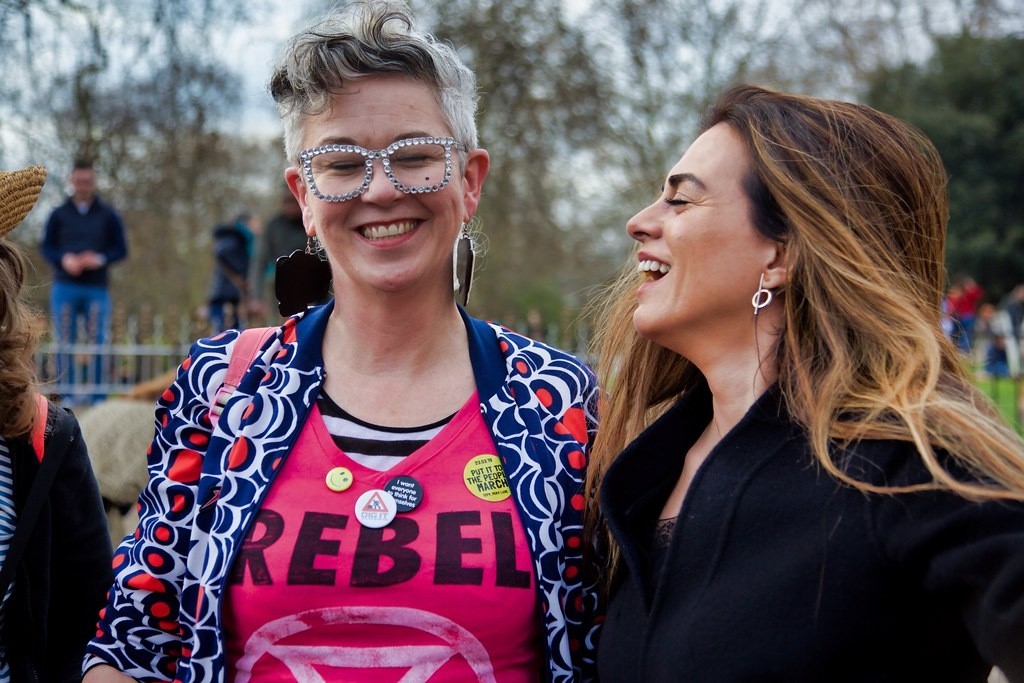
[[207, 212, 259, 332], [942, 275, 1024, 422], [80, 0, 609, 682], [595, 84, 1024, 683], [41, 159, 127, 410], [252, 183, 309, 323], [0, 236, 115, 683]]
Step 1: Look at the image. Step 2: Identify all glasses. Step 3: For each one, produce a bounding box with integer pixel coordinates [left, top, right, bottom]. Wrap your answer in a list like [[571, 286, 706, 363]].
[[300, 136, 466, 203]]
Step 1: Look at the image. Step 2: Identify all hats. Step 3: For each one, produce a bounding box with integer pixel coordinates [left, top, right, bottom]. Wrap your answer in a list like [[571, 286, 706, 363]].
[[1, 165, 47, 239]]
